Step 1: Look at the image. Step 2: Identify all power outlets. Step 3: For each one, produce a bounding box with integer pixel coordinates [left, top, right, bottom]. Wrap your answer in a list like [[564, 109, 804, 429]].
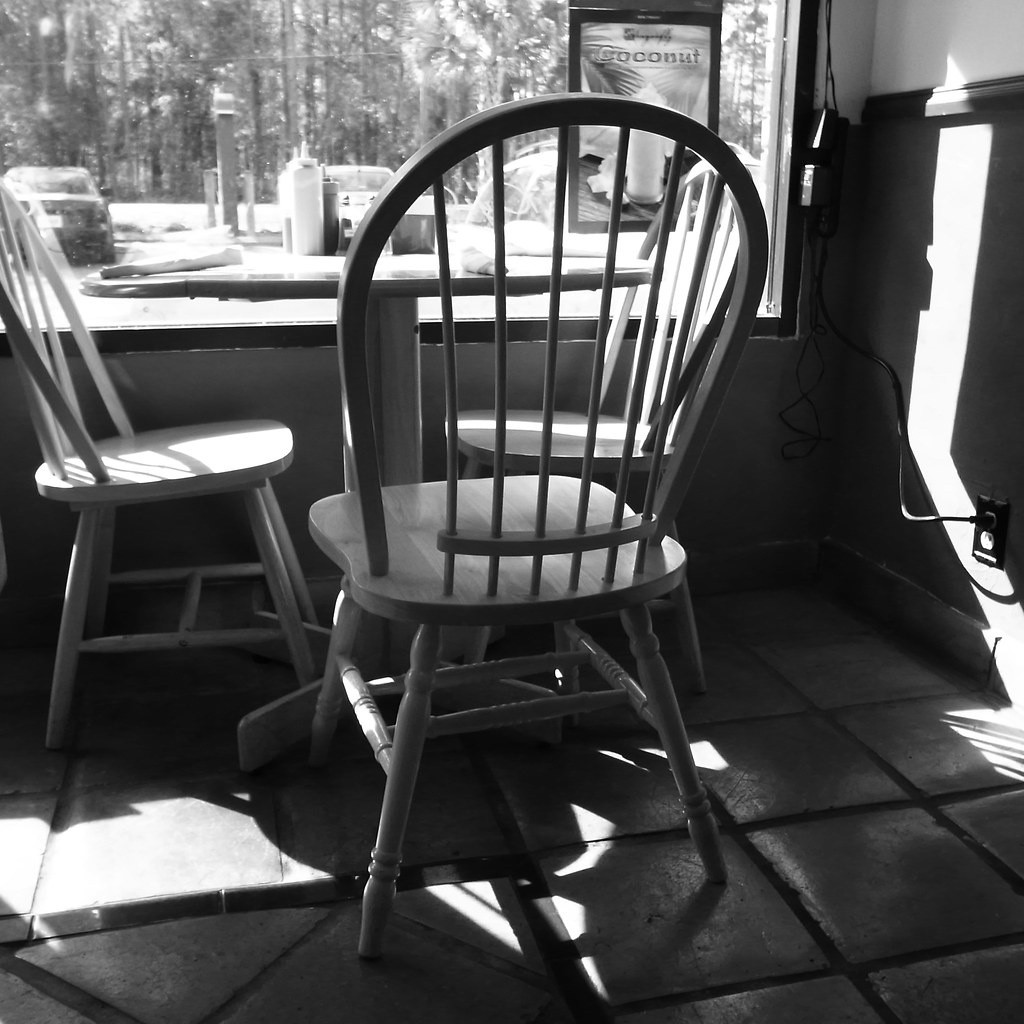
[[972, 494, 1011, 569]]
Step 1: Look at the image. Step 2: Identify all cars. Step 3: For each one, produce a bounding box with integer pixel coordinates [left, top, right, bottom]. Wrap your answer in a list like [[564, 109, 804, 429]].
[[322, 163, 397, 252], [3, 165, 117, 267]]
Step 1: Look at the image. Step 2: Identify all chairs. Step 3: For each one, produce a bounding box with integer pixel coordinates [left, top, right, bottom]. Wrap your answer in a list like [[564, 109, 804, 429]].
[[456, 155, 739, 730], [0, 161, 318, 746], [309, 91, 728, 956]]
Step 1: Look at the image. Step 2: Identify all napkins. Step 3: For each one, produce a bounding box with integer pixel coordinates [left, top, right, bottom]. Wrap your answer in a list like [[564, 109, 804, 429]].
[[463, 247, 508, 274], [100, 248, 243, 278]]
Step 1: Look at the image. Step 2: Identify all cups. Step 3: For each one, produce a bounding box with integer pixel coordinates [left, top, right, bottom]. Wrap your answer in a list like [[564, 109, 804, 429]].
[[625, 92, 668, 204]]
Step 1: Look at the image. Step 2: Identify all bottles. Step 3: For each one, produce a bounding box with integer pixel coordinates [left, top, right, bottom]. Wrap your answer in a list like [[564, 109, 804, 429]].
[[291, 140, 325, 256], [277, 160, 293, 254], [322, 163, 338, 256]]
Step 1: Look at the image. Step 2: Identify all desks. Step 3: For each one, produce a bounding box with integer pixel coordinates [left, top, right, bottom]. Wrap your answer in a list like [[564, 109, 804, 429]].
[[102, 259, 650, 773]]
